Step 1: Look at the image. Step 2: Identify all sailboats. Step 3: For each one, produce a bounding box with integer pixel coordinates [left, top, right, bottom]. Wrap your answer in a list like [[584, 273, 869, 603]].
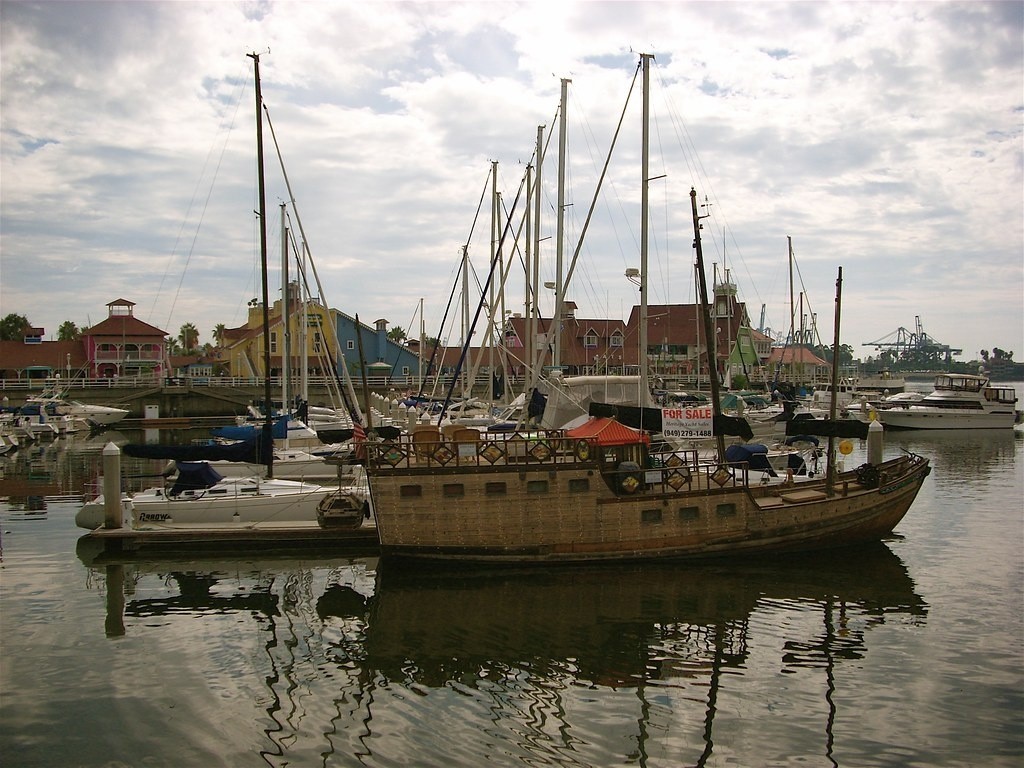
[[1, 48, 936, 565]]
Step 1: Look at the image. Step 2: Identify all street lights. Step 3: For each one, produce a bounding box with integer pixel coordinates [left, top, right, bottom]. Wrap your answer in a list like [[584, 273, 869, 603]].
[[66, 352, 72, 385], [237, 353, 241, 387]]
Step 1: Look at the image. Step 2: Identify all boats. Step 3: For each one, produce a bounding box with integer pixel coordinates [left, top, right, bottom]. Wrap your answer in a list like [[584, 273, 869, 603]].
[[875, 374, 1021, 429]]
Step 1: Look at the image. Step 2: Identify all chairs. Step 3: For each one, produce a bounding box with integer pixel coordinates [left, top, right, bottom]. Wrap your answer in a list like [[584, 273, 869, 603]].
[[412, 425, 480, 464]]
